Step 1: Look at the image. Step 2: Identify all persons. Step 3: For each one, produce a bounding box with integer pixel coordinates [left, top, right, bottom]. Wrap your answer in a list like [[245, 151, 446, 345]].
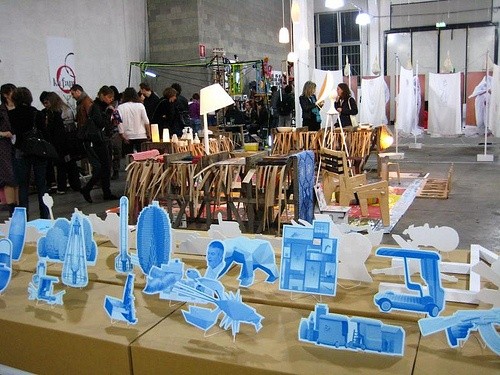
[[205, 240, 226, 279], [0, 82, 294, 222], [299, 80, 322, 130], [334, 82, 358, 127]]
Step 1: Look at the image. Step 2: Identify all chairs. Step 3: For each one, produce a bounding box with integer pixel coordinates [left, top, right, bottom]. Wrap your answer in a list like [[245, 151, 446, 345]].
[[320, 147, 390, 227]]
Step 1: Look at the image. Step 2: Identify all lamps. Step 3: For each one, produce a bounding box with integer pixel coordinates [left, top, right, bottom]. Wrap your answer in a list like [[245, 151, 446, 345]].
[[200, 83, 235, 154], [279, 0, 371, 62]]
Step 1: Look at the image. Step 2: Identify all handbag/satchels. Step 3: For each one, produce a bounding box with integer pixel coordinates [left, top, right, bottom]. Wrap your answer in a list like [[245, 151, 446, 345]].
[[347, 97, 359, 127], [24, 141, 58, 169]]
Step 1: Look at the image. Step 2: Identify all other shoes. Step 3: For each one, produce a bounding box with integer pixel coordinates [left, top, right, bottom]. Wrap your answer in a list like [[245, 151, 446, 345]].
[[80, 187, 93, 204], [102, 193, 121, 201], [8, 203, 17, 217]]
